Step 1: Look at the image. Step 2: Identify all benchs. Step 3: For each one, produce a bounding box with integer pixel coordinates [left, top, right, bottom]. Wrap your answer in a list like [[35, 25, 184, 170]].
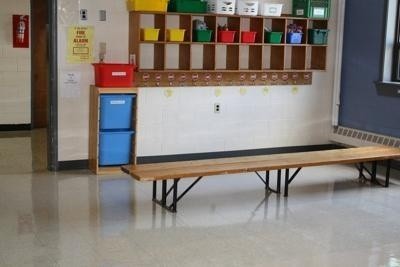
[[120, 144, 400, 214]]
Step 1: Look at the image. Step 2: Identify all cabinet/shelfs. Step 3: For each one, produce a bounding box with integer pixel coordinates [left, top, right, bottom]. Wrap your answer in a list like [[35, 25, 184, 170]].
[[90, 171, 137, 242], [128, 10, 331, 73], [89, 85, 139, 175]]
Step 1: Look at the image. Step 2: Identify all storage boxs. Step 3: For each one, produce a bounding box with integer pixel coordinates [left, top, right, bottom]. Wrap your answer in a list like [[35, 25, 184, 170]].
[[217, 30, 237, 43], [192, 29, 213, 42], [101, 179, 133, 207], [258, 2, 283, 17], [166, 27, 186, 41], [207, 0, 236, 14], [168, 0, 209, 13], [100, 94, 136, 129], [264, 30, 283, 43], [235, 0, 259, 16], [140, 26, 160, 41], [126, 0, 171, 13], [98, 131, 135, 167], [307, 28, 330, 45], [292, 0, 331, 19], [241, 31, 257, 43], [101, 209, 130, 236], [286, 31, 304, 43], [91, 62, 138, 87]]
